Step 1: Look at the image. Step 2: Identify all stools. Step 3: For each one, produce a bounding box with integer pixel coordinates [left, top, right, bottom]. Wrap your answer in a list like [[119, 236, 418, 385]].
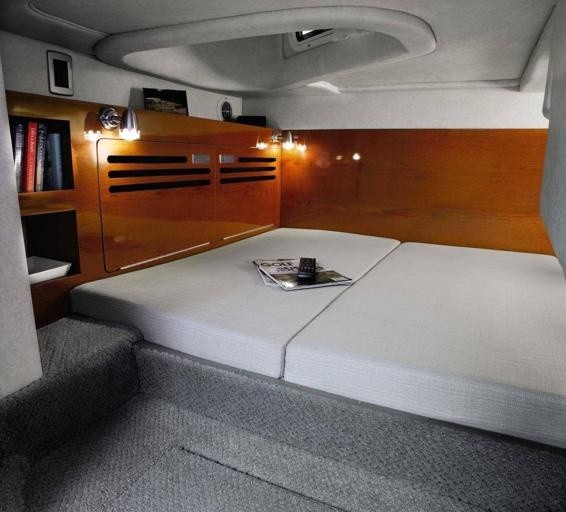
[[0, 317, 138, 461]]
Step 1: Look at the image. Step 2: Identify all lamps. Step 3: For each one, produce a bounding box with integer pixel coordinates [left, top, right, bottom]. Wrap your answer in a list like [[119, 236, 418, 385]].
[[100, 105, 140, 141], [271, 130, 294, 149]]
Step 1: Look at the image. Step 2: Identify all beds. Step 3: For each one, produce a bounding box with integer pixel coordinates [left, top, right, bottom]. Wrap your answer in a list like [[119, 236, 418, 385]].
[[69, 226, 566, 511]]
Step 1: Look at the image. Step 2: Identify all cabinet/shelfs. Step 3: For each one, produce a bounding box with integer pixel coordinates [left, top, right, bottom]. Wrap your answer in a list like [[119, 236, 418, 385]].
[[7, 113, 81, 286]]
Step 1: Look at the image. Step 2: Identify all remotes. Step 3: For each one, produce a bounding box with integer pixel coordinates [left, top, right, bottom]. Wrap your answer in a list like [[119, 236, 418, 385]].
[[297, 258, 316, 282]]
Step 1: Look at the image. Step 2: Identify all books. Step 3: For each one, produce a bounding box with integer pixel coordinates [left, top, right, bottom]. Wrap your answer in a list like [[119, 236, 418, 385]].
[[253, 258, 353, 292], [13, 121, 64, 193]]
